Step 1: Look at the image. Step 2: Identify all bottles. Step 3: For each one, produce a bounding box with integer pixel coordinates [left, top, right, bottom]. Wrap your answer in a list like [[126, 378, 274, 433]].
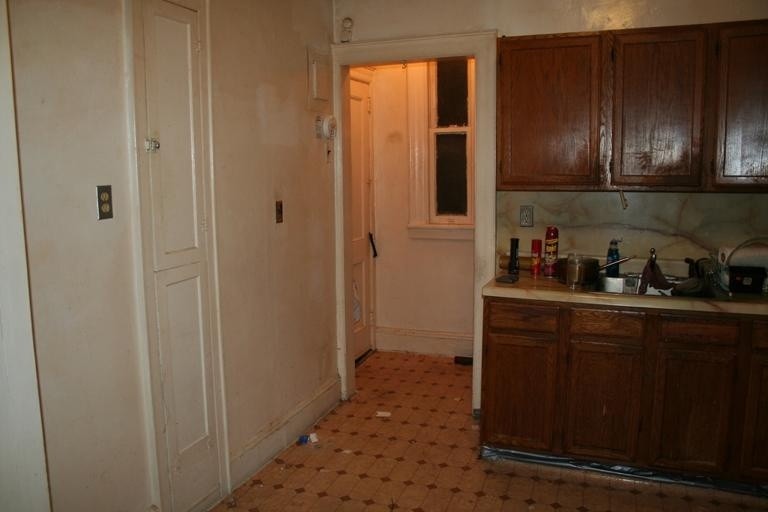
[[605, 238, 619, 278]]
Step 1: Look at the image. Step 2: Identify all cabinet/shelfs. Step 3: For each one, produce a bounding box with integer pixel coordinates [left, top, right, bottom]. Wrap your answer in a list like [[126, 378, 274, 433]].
[[497, 18, 768, 190], [483, 297, 768, 496]]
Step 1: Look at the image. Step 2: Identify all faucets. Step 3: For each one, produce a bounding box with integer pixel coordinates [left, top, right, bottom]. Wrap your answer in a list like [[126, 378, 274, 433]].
[[648, 248, 657, 269], [591, 255, 637, 276]]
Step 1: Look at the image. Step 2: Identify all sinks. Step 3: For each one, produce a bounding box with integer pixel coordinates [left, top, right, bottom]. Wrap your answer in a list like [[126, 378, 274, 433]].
[[586, 273, 719, 299]]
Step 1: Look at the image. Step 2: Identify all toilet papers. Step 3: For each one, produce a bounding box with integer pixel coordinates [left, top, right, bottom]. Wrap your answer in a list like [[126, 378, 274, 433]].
[[718, 246, 768, 268]]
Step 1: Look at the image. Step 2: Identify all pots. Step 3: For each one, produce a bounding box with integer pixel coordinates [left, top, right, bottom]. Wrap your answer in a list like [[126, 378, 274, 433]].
[[557, 254, 636, 284]]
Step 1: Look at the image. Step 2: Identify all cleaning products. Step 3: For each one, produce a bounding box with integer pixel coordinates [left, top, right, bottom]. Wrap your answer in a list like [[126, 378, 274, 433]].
[[606, 239, 622, 276]]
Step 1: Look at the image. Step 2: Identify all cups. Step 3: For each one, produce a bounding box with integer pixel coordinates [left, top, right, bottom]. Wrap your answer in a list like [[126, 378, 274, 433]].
[[606, 277, 625, 294], [566, 253, 584, 292]]
